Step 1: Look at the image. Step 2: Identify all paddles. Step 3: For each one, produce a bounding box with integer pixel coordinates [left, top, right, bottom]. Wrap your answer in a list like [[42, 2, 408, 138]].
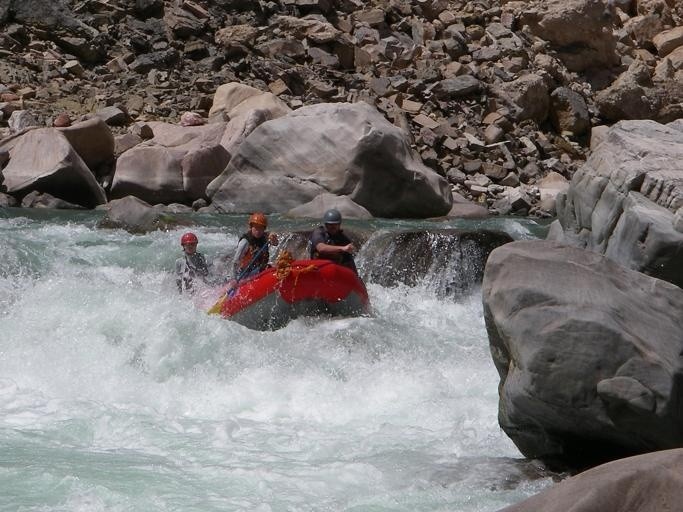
[[208, 238, 271, 315]]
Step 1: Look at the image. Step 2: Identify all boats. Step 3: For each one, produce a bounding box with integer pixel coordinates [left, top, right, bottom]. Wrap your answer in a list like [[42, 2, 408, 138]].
[[204, 257, 371, 332]]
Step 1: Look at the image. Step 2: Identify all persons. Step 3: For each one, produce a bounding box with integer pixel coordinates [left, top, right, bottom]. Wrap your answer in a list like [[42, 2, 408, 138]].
[[173, 233, 210, 297], [228, 211, 279, 292], [307, 208, 359, 274]]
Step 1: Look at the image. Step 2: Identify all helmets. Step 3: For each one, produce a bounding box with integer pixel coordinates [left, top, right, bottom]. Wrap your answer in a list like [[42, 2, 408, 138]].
[[324, 210, 342, 224], [181, 233, 198, 244], [249, 214, 266, 226]]
